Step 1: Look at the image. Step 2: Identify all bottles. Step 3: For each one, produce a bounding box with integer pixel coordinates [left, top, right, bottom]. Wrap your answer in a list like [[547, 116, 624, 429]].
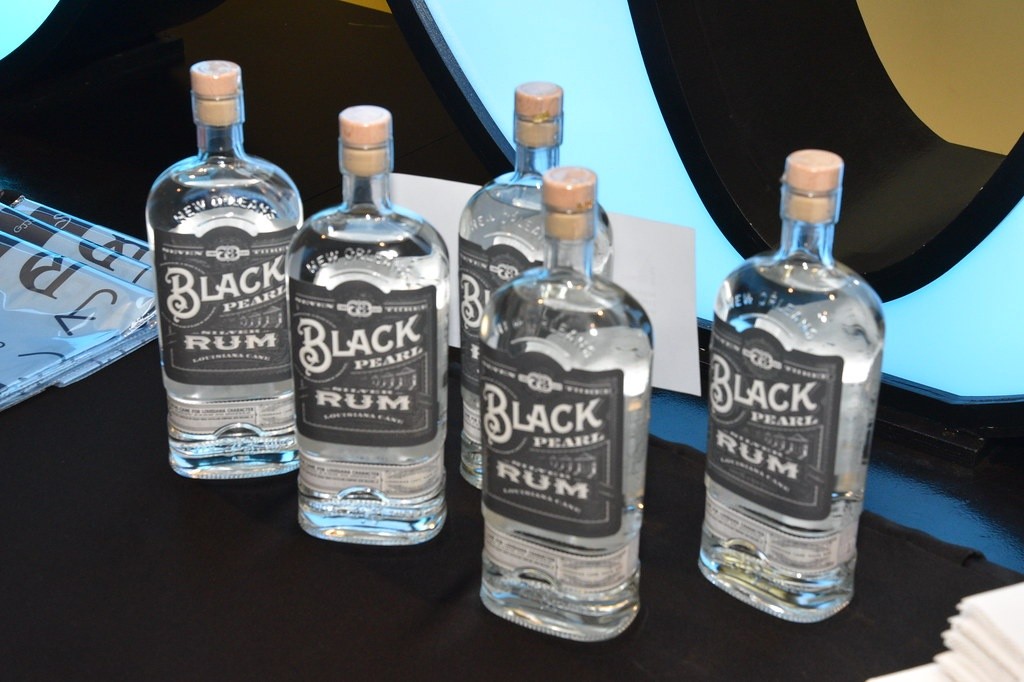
[[145, 59, 304, 480], [284, 104, 450, 547], [696, 149, 886, 625], [479, 166, 654, 642], [458, 80, 615, 491]]
